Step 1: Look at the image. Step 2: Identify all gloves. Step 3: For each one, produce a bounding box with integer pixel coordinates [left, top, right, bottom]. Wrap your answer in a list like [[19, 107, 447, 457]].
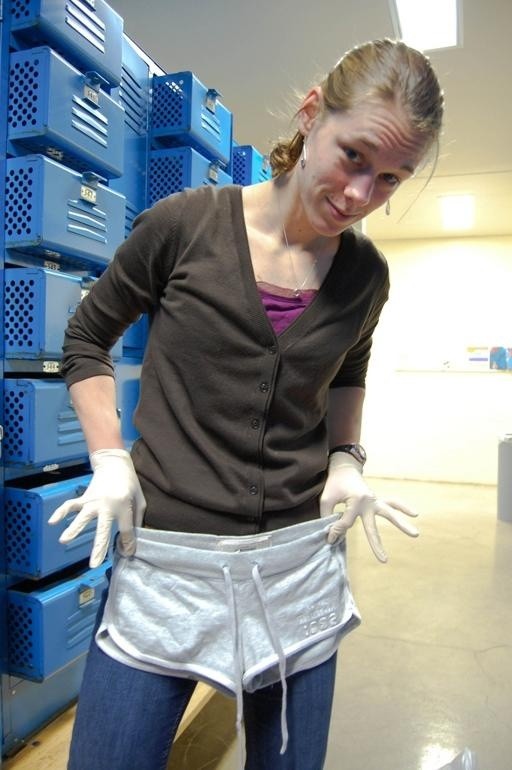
[[49, 448, 147, 568], [319, 452, 418, 562]]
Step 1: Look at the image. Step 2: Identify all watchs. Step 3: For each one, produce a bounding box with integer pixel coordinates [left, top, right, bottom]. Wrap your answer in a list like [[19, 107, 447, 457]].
[[328, 442, 366, 465]]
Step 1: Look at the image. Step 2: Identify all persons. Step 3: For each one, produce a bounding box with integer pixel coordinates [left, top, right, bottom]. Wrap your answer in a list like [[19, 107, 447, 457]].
[[47, 40, 445, 770]]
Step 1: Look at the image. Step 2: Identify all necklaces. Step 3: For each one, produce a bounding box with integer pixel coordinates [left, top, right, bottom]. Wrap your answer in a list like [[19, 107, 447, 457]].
[[272, 183, 329, 297]]
